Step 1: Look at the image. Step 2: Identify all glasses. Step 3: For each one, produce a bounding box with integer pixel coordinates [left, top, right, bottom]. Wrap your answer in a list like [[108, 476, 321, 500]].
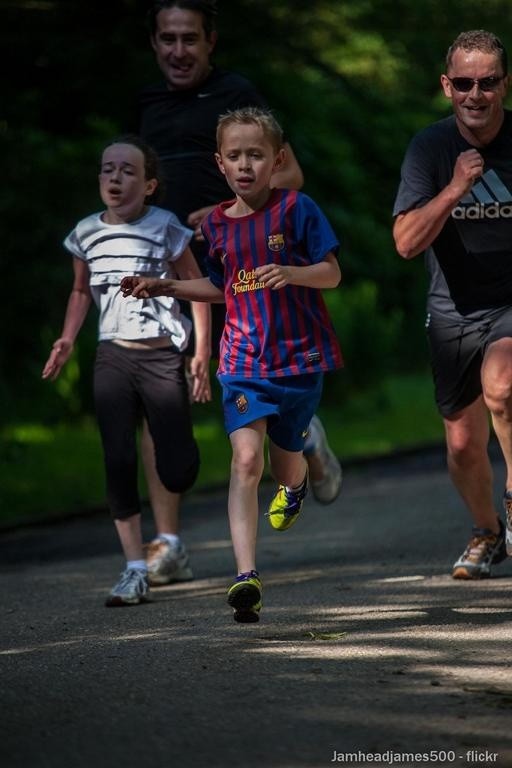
[[446, 73, 506, 91]]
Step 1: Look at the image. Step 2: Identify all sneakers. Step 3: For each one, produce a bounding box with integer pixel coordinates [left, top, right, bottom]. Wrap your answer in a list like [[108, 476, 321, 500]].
[[453, 516, 508, 580], [503, 487, 512, 557], [145, 539, 192, 585], [228, 571, 261, 622], [303, 415, 341, 505], [267, 461, 310, 530], [106, 571, 151, 606]]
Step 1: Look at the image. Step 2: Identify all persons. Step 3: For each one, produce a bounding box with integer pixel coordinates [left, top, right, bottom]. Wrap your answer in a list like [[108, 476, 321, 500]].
[[390, 25, 512, 580], [119, 107, 345, 629], [112, 3, 345, 594], [34, 139, 212, 605]]
